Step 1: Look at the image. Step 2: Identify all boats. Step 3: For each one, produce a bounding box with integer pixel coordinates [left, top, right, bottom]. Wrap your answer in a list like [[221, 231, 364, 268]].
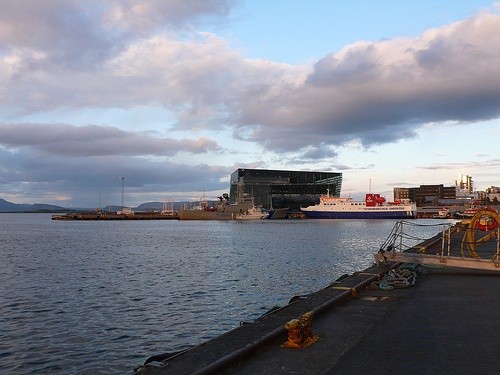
[[237, 208, 269, 221], [301, 194, 417, 219]]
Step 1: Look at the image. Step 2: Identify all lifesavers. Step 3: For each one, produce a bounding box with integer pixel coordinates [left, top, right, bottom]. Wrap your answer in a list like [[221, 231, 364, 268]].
[[474, 207, 498, 231]]
